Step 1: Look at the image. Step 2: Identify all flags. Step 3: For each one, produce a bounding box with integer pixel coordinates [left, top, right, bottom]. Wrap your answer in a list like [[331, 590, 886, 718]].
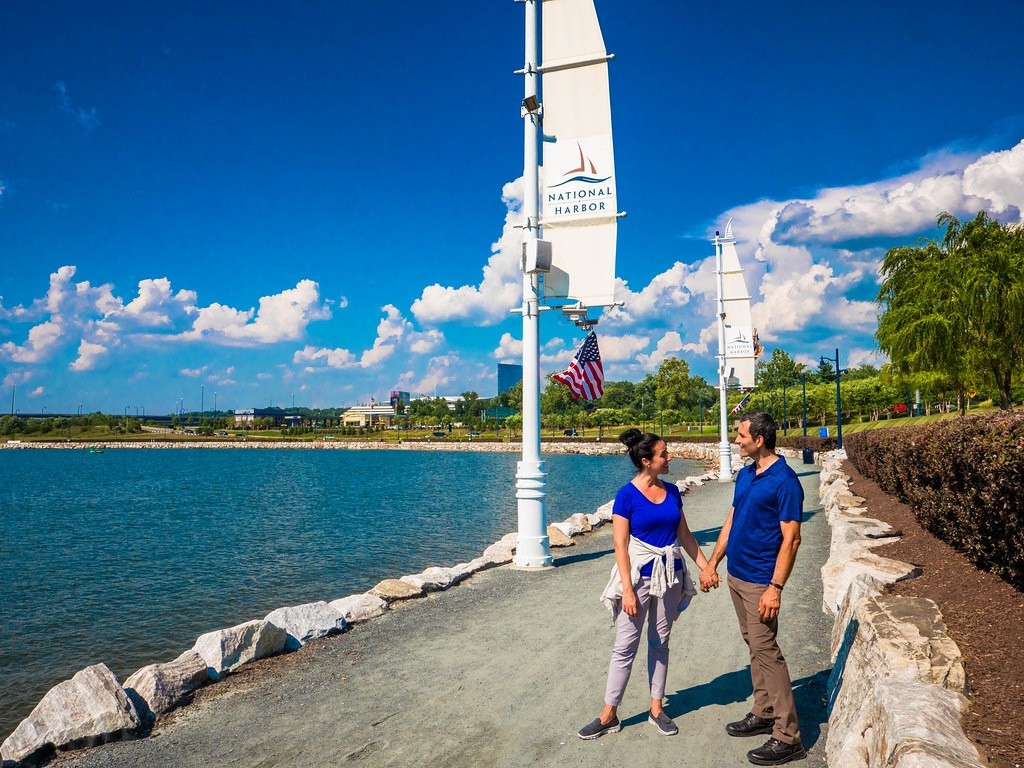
[[551, 329, 604, 400], [732, 397, 749, 414]]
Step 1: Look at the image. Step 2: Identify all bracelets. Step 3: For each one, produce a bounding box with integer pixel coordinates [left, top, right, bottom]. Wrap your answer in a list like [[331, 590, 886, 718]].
[[770, 581, 783, 590]]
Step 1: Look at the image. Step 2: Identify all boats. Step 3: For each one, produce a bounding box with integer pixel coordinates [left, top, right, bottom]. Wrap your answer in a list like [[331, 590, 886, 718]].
[[90, 447, 104, 454]]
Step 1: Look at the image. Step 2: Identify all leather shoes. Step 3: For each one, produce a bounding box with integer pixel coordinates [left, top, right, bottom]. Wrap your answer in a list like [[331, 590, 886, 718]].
[[726, 711, 774, 737], [747, 736, 808, 765]]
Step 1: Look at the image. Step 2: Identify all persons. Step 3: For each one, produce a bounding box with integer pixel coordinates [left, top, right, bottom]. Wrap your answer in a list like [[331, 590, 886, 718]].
[[577, 427, 722, 741], [699, 411, 809, 765]]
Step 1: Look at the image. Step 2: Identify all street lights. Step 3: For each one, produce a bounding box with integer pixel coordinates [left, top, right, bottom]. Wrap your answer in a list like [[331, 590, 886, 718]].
[[791, 374, 806, 437], [134, 407, 138, 415], [181, 398, 183, 414], [201, 384, 204, 416], [711, 217, 758, 483], [78, 405, 82, 415], [125, 406, 130, 415], [512, 1, 627, 570], [818, 348, 848, 449], [42, 407, 46, 418], [141, 406, 144, 415], [214, 392, 217, 417]]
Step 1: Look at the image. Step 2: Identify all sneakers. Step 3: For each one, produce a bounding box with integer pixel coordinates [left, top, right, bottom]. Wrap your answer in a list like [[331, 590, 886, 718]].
[[648, 708, 678, 736], [578, 715, 621, 740]]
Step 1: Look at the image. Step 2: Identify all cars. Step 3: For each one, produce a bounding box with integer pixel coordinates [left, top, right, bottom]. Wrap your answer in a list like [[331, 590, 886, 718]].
[[893, 401, 919, 414], [220, 431, 228, 435], [465, 432, 480, 436], [235, 434, 245, 437], [184, 430, 193, 433], [564, 430, 579, 436], [325, 435, 336, 438], [433, 432, 448, 437]]
